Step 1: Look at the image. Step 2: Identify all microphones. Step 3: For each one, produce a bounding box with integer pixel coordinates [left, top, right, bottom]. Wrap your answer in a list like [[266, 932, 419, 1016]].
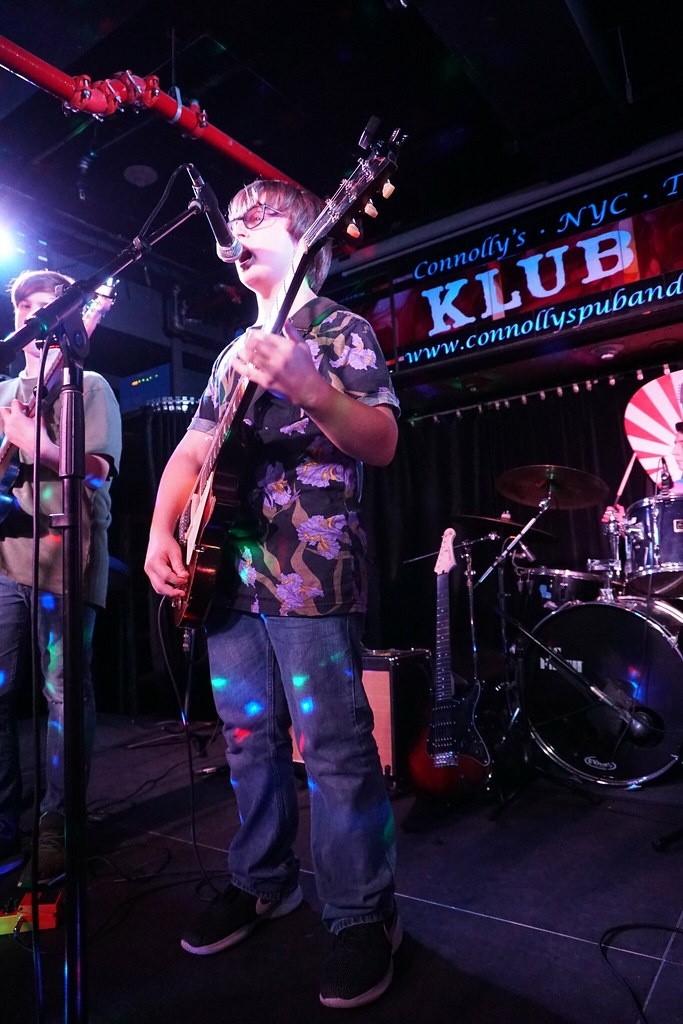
[[628, 711, 653, 737], [187, 163, 243, 263], [519, 540, 536, 562], [662, 458, 674, 489]]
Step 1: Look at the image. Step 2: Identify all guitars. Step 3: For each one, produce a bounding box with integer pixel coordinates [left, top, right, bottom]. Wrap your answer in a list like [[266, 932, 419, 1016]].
[[1, 273, 118, 489], [167, 119, 410, 631]]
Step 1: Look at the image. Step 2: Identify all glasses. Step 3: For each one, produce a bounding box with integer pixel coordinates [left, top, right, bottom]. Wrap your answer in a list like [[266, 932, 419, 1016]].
[[225, 204, 288, 234]]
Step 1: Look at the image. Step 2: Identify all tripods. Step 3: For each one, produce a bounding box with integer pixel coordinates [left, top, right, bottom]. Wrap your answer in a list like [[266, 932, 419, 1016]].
[[475, 479, 555, 729]]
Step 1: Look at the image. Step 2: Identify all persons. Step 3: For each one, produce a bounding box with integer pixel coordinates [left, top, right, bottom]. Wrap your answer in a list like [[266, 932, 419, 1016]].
[[144, 176, 399, 1006], [0, 269, 123, 888]]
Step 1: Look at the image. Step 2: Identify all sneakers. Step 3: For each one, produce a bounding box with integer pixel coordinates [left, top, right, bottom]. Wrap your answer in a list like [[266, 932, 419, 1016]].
[[1, 840, 23, 871], [318, 903, 402, 1008], [20, 832, 78, 890], [179, 877, 302, 955]]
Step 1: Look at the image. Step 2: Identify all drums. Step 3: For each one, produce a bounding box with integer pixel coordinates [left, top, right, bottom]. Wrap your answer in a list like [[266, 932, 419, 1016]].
[[522, 596, 683, 787], [519, 566, 603, 609], [628, 493, 683, 586]]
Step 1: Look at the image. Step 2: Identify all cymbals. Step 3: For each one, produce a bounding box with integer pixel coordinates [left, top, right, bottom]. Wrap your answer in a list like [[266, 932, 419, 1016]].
[[453, 510, 549, 539], [495, 462, 610, 510]]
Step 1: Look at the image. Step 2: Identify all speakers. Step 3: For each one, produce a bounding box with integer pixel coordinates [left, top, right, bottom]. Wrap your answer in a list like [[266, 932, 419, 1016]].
[[289, 650, 438, 793]]
